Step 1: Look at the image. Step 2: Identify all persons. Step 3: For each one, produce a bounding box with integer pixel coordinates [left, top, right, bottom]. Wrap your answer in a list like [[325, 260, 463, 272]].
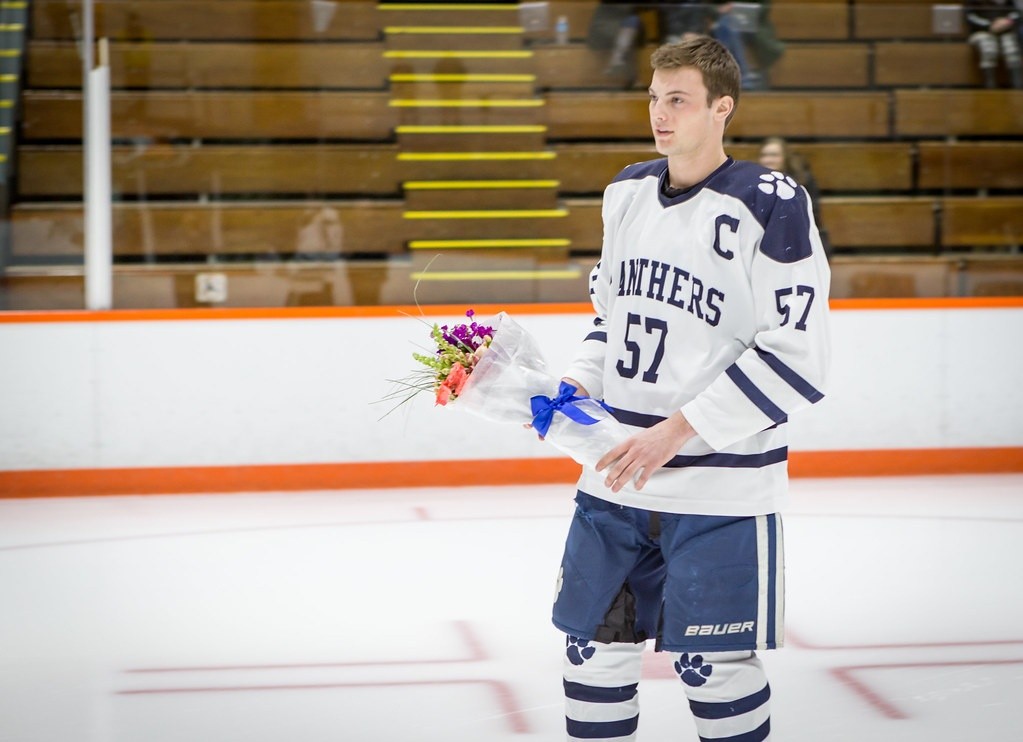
[[522, 36, 834, 742], [586, 0, 832, 259], [963, 0, 1023, 90]]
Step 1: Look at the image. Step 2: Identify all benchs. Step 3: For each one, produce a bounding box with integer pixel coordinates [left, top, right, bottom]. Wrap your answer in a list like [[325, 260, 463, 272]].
[[0, 0, 1023, 315]]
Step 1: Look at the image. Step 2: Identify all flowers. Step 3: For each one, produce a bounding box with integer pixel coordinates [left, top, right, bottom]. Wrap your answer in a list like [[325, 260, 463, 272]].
[[368, 249, 635, 479]]
